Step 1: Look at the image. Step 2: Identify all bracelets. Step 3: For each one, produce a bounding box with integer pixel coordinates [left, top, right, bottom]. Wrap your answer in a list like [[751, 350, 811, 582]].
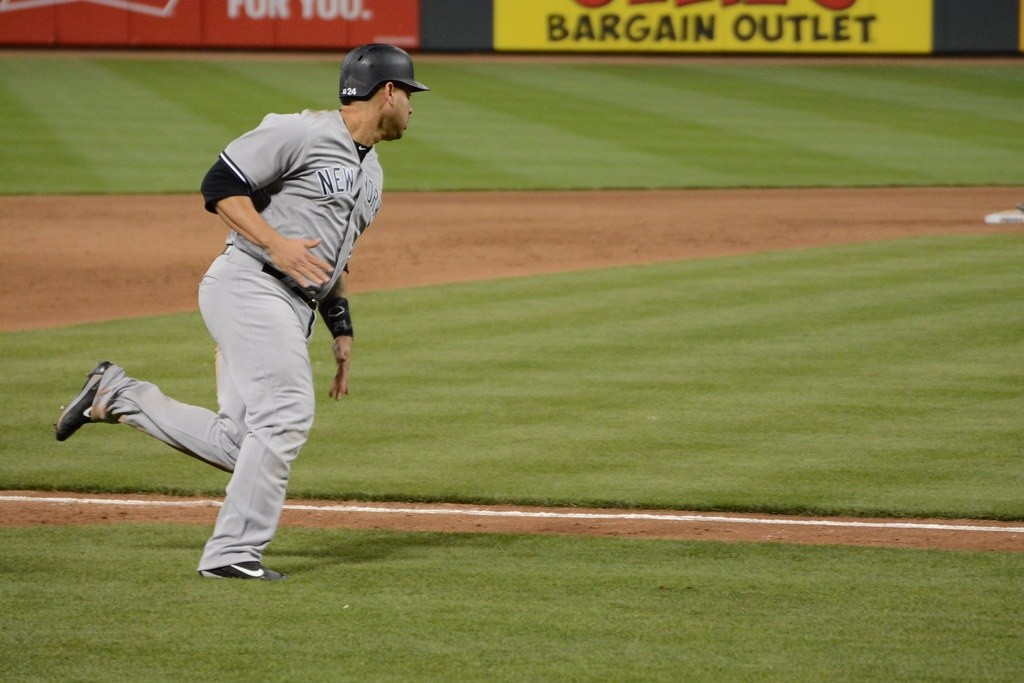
[[318, 296, 353, 339]]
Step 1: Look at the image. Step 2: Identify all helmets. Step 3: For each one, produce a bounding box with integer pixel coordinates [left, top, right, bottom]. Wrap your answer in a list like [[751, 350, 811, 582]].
[[339, 43, 431, 99]]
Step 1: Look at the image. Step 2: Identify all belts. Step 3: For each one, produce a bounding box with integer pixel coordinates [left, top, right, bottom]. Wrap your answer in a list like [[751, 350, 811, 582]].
[[221, 244, 317, 310]]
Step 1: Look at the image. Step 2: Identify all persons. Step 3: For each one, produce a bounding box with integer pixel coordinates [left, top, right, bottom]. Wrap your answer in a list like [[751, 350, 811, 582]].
[[55, 43, 430, 582]]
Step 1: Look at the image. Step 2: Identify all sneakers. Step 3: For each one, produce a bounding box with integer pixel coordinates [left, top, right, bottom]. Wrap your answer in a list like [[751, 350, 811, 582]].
[[51, 360, 114, 441], [198, 561, 287, 581]]
